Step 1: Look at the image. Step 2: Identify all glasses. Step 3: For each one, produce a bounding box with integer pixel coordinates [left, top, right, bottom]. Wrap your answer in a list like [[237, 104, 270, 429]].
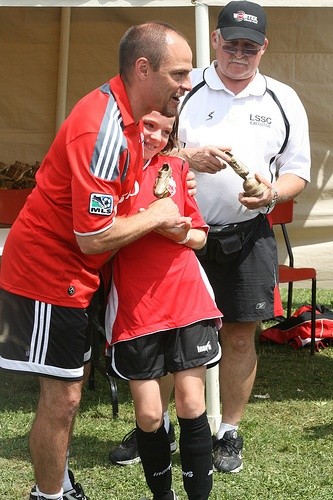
[[219, 35, 260, 58]]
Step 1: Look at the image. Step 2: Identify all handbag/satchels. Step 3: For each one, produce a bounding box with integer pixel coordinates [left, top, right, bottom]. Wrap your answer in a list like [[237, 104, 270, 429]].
[[191, 233, 244, 265]]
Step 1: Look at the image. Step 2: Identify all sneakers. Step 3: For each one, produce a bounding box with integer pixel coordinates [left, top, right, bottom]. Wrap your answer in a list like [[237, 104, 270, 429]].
[[108, 421, 177, 465], [212, 428, 244, 474], [28, 468, 90, 500]]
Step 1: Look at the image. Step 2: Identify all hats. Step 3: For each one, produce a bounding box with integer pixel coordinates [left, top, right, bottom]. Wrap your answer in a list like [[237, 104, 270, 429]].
[[216, 0, 267, 46]]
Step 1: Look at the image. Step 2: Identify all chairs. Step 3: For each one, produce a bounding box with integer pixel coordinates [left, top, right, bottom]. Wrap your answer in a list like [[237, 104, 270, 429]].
[[271, 200, 317, 356]]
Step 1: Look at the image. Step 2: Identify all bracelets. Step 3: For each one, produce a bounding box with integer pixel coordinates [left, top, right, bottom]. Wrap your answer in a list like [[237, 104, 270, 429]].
[[175, 231, 190, 244]]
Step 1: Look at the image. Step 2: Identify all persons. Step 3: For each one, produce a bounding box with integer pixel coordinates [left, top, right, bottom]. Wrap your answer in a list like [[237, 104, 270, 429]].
[[100, 111, 224, 500], [108, 0, 311, 471], [0, 21, 193, 500]]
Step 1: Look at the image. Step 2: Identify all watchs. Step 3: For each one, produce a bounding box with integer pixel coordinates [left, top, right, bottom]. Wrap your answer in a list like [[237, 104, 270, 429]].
[[264, 186, 278, 208]]
